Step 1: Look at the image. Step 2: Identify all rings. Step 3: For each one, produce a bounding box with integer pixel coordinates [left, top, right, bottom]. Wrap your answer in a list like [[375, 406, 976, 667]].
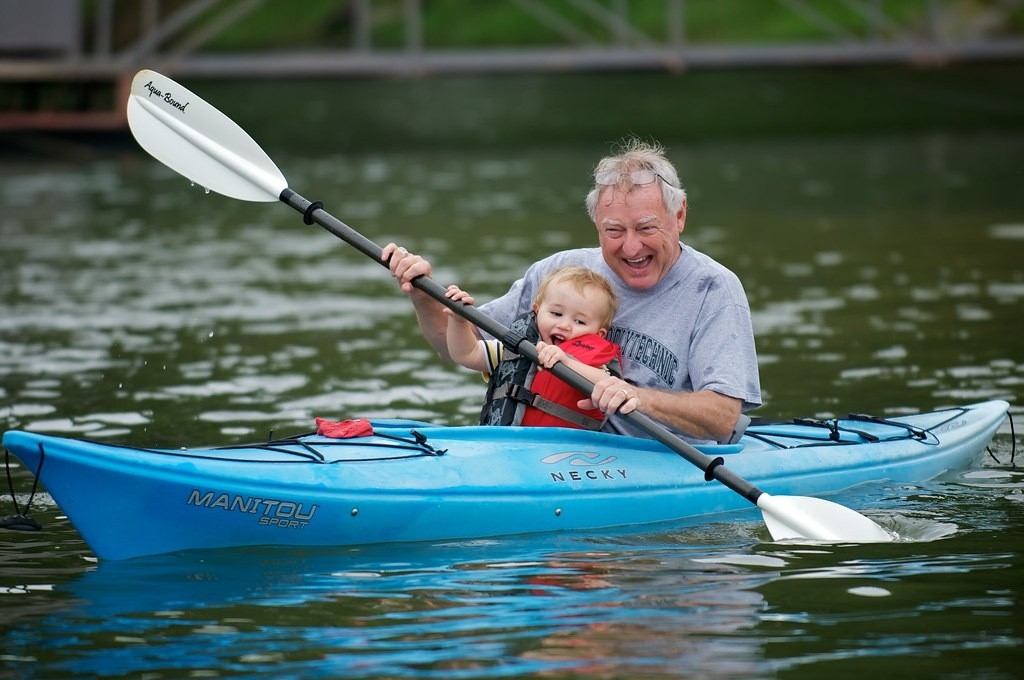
[[399, 246, 408, 256], [622, 389, 629, 397]]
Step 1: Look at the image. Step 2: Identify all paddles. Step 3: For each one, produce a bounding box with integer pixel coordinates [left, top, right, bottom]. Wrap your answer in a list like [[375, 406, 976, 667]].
[[126, 69, 895, 542]]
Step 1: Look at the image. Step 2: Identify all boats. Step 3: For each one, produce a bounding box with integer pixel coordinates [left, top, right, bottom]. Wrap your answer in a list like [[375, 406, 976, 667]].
[[1, 398, 1016, 562]]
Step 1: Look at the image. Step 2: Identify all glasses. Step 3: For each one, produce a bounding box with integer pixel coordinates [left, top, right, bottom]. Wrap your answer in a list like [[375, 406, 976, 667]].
[[593, 168, 675, 188]]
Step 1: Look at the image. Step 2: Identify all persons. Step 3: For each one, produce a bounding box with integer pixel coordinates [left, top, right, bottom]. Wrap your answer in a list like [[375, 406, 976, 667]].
[[381, 137, 764, 446], [441, 264, 624, 428]]
